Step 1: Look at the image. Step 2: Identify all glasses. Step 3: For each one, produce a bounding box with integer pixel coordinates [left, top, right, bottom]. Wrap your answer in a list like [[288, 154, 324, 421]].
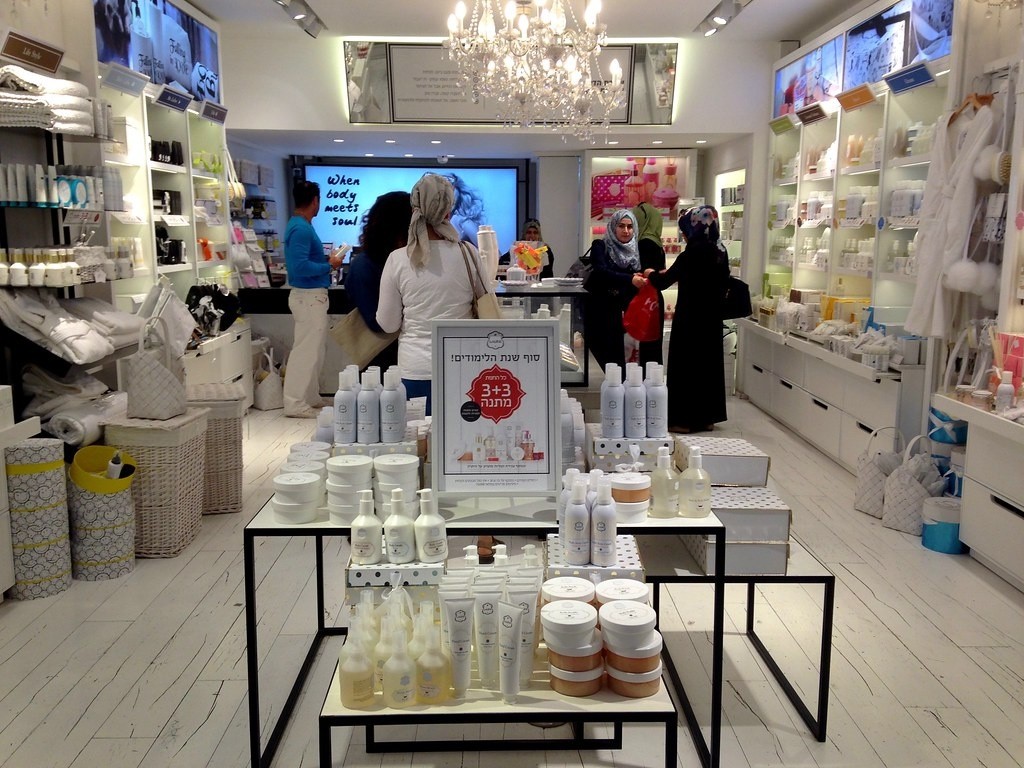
[[523, 217, 541, 227], [637, 201, 648, 220]]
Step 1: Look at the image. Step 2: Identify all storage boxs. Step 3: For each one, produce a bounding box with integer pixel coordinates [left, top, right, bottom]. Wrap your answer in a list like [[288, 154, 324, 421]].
[[763, 271, 792, 297], [675, 466, 792, 541], [673, 434, 772, 488], [348, 534, 444, 586], [678, 532, 790, 577]]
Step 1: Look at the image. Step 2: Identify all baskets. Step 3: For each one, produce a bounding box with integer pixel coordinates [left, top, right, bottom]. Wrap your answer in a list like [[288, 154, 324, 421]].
[[105, 382, 243, 560]]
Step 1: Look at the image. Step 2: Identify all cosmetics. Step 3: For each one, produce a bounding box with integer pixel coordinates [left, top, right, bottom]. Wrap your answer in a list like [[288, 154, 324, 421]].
[[156, 236, 187, 264], [591, 155, 682, 254], [780, 47, 833, 116], [538, 303, 584, 372], [476, 224, 499, 282], [955, 331, 1024, 427], [149, 134, 184, 167], [270, 361, 794, 713], [0, 163, 123, 211], [154, 190, 181, 215], [95, 0, 218, 103], [678, 117, 938, 372], [0, 218, 144, 286]]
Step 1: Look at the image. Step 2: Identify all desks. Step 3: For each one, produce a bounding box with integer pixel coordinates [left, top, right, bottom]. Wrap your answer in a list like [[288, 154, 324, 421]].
[[242, 423, 834, 768]]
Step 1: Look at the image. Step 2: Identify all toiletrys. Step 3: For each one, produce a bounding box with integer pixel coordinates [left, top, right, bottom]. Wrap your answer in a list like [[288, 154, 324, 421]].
[[276, 366, 710, 711], [749, 121, 934, 375]]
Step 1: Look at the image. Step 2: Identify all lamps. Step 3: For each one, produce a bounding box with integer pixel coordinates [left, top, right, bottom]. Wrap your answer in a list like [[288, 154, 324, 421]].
[[273, 0, 324, 40], [701, 0, 742, 36], [448, 0, 626, 148]]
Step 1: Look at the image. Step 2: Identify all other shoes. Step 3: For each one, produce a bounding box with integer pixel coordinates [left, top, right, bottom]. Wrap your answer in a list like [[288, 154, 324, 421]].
[[286, 406, 321, 419], [313, 399, 333, 409], [667, 424, 714, 433]]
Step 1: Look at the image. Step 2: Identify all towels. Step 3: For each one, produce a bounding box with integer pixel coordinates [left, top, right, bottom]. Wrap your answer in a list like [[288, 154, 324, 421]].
[[0, 288, 144, 367], [23, 369, 128, 443], [0, 65, 95, 138]]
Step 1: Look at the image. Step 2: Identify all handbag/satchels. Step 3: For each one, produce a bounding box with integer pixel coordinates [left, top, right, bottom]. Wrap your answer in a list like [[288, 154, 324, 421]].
[[329, 306, 402, 373], [623, 273, 660, 343], [458, 239, 502, 319], [853, 426, 906, 519], [126, 317, 188, 418], [253, 347, 289, 411], [881, 434, 949, 536], [723, 275, 754, 320], [559, 246, 593, 309]]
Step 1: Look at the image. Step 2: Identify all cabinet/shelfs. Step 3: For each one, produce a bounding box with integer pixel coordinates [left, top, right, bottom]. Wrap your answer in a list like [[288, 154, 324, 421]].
[[494, 281, 607, 410], [645, 44, 670, 123], [349, 42, 374, 123], [0, 52, 278, 601], [662, 53, 1024, 592]]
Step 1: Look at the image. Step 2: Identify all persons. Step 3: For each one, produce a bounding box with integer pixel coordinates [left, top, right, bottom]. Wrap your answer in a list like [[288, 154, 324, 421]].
[[499, 219, 554, 318], [421, 173, 501, 256], [632, 203, 666, 381], [282, 181, 347, 418], [582, 208, 666, 383], [376, 172, 492, 416], [345, 191, 412, 386], [645, 205, 728, 434]]
